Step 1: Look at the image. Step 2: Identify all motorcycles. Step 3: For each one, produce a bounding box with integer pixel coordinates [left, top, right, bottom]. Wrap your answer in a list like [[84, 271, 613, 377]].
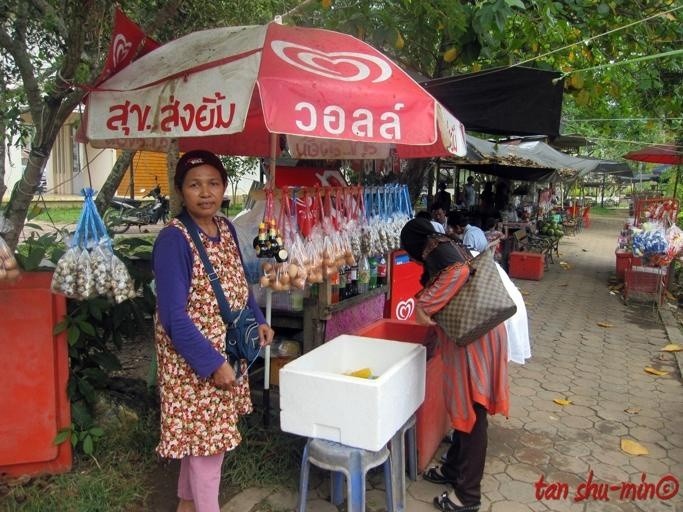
[[104, 175, 173, 232]]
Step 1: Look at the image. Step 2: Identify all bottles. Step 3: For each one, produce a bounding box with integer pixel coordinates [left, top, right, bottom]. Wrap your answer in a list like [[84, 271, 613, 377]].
[[307, 252, 386, 305]]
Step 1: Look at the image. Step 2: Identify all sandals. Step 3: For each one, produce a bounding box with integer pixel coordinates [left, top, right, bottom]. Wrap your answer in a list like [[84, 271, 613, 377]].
[[423, 466, 447, 484], [434, 491, 481, 512]]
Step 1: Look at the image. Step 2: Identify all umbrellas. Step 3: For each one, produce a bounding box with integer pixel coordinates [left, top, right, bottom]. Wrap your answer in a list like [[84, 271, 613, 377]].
[[75, 14, 469, 427], [622, 143, 683, 199]]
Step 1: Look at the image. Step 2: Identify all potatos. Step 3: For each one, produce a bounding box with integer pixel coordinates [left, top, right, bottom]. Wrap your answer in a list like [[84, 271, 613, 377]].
[[257, 248, 357, 292]]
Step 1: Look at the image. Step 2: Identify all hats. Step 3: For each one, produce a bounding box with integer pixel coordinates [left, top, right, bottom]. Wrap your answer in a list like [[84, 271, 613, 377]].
[[175, 149, 227, 189]]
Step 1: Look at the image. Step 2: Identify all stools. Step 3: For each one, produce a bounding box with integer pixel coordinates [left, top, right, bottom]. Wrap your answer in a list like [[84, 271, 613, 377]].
[[298, 415, 418, 512]]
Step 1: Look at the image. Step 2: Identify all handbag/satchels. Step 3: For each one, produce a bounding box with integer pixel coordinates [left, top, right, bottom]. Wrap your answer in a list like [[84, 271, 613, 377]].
[[414, 250, 517, 348], [226, 309, 260, 362]]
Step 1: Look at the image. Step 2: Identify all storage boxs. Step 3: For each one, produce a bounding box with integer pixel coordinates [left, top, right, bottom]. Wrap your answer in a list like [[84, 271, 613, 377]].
[[616, 249, 640, 276], [508, 251, 545, 281], [278, 334, 427, 452]]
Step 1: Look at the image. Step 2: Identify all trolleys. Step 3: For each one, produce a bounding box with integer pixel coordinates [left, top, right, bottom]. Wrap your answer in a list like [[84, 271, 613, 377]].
[[621, 253, 667, 312]]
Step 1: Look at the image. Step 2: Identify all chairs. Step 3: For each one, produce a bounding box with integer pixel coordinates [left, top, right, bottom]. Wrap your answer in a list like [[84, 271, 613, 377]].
[[561, 211, 582, 237], [513, 229, 559, 268]]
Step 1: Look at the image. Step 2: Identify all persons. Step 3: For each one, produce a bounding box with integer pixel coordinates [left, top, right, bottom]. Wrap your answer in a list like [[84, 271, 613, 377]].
[[154, 148, 275, 512], [414, 173, 498, 253], [399, 218, 517, 511], [441, 250, 532, 463]]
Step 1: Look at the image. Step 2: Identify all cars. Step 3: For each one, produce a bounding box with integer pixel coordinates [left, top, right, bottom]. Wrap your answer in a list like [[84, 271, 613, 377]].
[[579, 190, 619, 208], [21, 165, 46, 194]]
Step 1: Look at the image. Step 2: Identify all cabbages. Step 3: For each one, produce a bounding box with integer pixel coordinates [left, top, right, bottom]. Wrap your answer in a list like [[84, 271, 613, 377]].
[[539, 222, 564, 242]]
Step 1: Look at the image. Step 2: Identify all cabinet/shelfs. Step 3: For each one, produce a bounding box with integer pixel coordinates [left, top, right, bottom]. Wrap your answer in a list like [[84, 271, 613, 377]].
[[245, 182, 416, 354]]
